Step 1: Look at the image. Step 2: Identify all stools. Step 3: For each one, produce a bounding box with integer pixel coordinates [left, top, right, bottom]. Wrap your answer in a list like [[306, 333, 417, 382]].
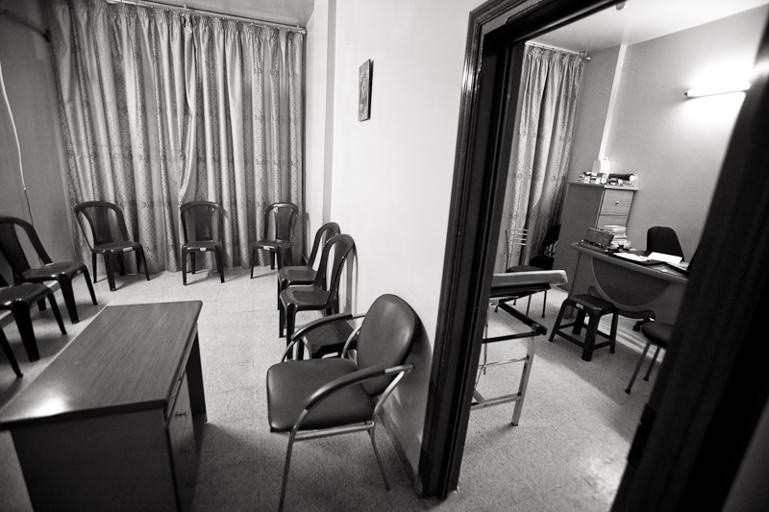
[[295, 321, 357, 364], [548, 292, 620, 361], [624, 320, 678, 393]]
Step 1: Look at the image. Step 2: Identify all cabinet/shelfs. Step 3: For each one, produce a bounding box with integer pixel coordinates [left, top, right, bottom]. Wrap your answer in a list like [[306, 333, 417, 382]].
[[552, 178, 639, 295]]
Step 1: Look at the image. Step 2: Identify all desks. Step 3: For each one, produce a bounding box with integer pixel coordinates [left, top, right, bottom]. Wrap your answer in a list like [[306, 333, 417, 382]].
[[4, 296, 212, 510], [470, 268, 568, 426], [564, 238, 692, 344]]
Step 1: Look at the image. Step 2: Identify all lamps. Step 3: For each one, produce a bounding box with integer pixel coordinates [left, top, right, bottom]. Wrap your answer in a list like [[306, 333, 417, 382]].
[[682, 80, 753, 100]]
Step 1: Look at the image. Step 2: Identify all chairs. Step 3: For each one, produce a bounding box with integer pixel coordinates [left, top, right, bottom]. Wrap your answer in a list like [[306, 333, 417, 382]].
[[0, 213, 98, 382], [494, 224, 553, 319], [71, 200, 152, 290], [266, 294, 420, 510], [177, 199, 229, 284], [279, 234, 355, 362], [276, 222, 340, 311], [644, 226, 685, 264], [251, 201, 298, 280]]
